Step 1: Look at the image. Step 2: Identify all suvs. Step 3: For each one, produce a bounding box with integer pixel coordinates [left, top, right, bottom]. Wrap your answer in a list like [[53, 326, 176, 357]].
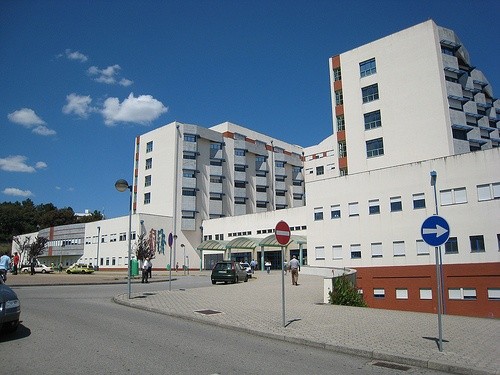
[[210, 260, 248, 284]]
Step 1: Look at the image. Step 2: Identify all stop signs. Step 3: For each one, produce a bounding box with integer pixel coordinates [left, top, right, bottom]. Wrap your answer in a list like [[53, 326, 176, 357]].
[[275, 221, 291, 245]]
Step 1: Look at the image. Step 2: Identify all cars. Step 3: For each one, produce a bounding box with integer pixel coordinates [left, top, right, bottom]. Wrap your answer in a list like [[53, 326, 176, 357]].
[[0, 272, 21, 333], [239, 263, 252, 278], [20, 263, 54, 274], [65, 264, 94, 274]]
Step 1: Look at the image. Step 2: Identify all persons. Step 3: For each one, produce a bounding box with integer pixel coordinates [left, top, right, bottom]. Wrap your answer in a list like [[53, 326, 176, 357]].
[[289, 255, 300, 286], [13, 252, 19, 275], [51, 261, 63, 272], [284, 260, 288, 275], [141, 257, 150, 283], [147, 259, 152, 278], [265, 258, 271, 274], [250, 259, 256, 274], [28, 255, 37, 275], [176, 263, 179, 272], [0, 250, 12, 284]]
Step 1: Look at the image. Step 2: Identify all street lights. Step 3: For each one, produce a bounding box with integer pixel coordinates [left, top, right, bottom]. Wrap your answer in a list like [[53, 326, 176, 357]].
[[114, 178, 133, 300], [169, 235, 178, 291], [429, 170, 443, 353], [181, 244, 187, 276]]
